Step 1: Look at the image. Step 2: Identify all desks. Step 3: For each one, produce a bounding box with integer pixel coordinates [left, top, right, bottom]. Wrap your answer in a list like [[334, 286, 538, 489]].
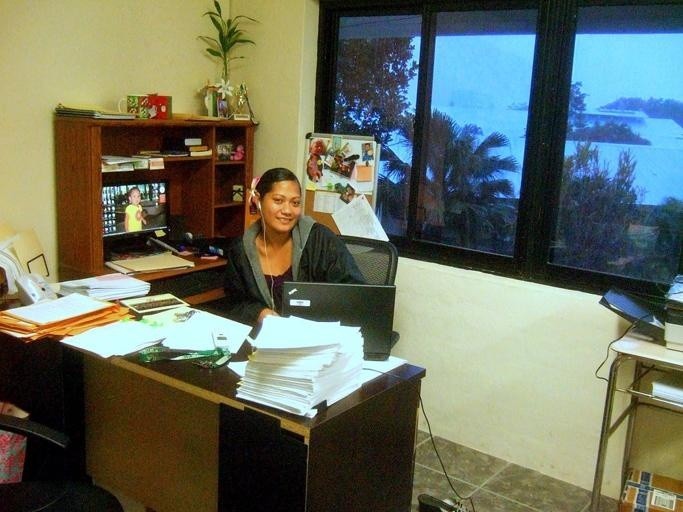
[[590, 327, 683, 511]]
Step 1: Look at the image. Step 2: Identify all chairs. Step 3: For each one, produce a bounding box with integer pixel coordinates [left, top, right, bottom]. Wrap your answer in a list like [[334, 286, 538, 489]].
[[335, 235, 397, 286], [1, 410, 123, 512]]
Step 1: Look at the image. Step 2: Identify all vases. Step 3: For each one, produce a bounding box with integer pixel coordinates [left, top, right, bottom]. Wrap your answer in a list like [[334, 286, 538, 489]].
[[218, 101, 228, 119]]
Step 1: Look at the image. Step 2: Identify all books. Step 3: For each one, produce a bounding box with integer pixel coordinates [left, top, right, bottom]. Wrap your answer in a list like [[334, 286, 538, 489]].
[[56, 107, 137, 120], [101, 136, 212, 165]]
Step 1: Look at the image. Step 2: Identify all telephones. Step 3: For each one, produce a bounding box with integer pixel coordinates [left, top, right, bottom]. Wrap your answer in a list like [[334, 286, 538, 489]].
[[14, 273, 58, 305]]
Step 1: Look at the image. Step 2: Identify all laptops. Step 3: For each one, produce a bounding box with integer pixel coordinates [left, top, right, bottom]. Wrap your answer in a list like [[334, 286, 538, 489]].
[[282, 281, 401, 361]]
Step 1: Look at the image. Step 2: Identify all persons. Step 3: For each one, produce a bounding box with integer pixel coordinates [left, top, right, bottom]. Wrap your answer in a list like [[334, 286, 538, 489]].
[[222, 167, 369, 323], [362, 143, 374, 161], [124, 187, 147, 232]]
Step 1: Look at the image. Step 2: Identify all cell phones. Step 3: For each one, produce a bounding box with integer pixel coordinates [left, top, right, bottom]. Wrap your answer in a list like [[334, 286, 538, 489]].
[[212, 330, 230, 349]]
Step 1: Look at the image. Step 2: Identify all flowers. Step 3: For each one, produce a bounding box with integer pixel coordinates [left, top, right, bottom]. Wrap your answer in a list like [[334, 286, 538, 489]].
[[198, 1, 263, 104]]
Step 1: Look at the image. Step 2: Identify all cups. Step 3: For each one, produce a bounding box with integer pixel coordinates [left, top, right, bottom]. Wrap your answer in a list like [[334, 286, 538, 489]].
[[118, 95, 172, 120]]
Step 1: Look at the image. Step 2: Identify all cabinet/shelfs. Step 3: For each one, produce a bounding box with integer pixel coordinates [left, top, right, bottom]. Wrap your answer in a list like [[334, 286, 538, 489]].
[[0, 296, 427, 511], [52, 114, 260, 305]]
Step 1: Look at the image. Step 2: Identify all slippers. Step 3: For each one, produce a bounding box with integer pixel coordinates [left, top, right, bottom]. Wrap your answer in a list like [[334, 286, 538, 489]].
[[418, 494, 467, 512]]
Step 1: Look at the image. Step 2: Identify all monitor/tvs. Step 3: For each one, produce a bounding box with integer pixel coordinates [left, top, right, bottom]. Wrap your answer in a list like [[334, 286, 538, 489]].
[[102, 179, 170, 254]]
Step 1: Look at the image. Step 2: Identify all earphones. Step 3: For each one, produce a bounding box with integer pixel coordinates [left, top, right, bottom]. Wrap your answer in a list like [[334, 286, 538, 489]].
[[257, 200, 262, 210]]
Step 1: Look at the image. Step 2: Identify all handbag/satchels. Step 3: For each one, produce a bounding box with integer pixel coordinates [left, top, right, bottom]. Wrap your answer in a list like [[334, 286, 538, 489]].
[[0, 402, 30, 485]]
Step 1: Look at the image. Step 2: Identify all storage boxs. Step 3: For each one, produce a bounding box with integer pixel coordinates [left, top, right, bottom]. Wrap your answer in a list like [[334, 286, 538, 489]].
[[617, 468, 683, 511]]
